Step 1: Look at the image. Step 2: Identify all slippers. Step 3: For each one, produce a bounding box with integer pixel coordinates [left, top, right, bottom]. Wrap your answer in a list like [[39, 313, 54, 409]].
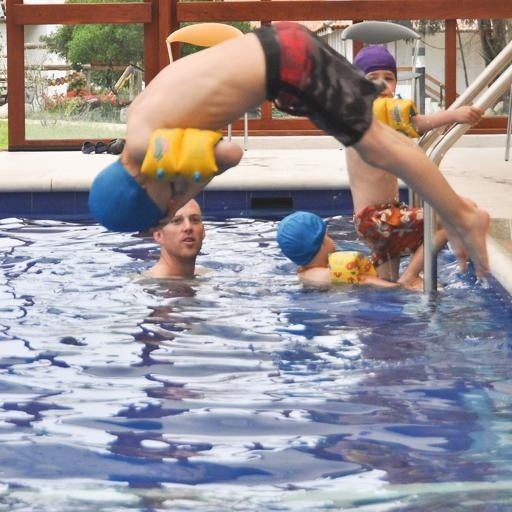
[[81, 138, 124, 155]]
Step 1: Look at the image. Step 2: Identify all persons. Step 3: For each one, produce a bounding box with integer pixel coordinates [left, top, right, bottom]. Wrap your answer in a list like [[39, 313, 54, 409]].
[[85, 23, 491, 282], [343, 43, 485, 292], [146, 199, 212, 278], [278, 209, 399, 288]]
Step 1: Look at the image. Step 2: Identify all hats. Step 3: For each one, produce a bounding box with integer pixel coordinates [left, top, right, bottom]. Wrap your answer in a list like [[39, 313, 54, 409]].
[[354, 45, 396, 80], [88, 159, 165, 233], [276, 211, 326, 265]]
